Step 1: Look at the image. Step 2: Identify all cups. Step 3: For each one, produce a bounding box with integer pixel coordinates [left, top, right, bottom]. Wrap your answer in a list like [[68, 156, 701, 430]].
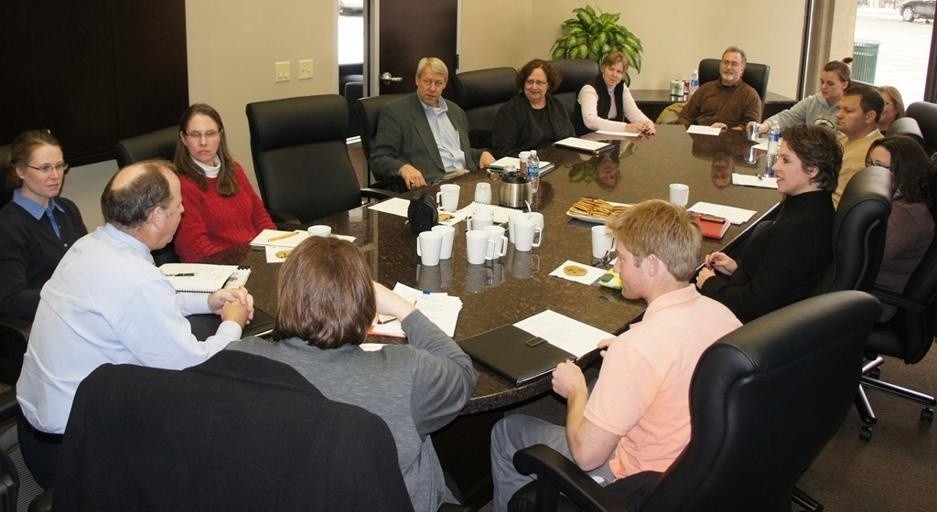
[[508, 214, 544, 252], [415, 261, 454, 295], [590, 225, 617, 259], [517, 151, 530, 171], [464, 258, 506, 295], [474, 183, 492, 204], [464, 225, 508, 266], [307, 224, 332, 238], [668, 183, 689, 208], [413, 224, 455, 268], [506, 245, 541, 281], [434, 182, 459, 211]]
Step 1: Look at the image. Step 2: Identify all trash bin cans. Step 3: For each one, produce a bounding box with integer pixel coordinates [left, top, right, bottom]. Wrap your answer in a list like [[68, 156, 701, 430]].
[[851, 39, 881, 83]]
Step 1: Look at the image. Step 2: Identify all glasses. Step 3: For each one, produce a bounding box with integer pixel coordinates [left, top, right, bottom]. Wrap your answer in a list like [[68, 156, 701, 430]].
[[26, 161, 69, 172], [885, 101, 895, 106], [866, 157, 889, 169], [527, 80, 547, 85], [187, 130, 217, 138]]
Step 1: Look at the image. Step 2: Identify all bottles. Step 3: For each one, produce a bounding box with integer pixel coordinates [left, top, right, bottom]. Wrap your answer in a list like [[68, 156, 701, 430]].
[[688, 70, 698, 96], [767, 120, 779, 154], [764, 155, 779, 178], [524, 149, 539, 194]]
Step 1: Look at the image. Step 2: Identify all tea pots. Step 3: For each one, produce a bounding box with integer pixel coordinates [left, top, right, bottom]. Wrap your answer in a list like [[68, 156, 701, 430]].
[[497, 172, 533, 209]]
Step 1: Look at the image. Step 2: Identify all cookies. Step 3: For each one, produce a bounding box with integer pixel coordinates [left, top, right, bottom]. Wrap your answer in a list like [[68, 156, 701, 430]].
[[569, 199, 626, 218], [276, 250, 289, 258], [564, 265, 587, 277]]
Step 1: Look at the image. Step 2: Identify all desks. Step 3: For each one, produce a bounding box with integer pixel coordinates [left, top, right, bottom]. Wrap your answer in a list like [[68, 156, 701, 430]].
[[186, 118, 790, 416], [630, 89, 799, 123]]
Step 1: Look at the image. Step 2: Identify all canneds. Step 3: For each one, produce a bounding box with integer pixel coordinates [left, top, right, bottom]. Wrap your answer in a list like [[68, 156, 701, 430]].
[[744, 144, 757, 164], [748, 124, 760, 141]]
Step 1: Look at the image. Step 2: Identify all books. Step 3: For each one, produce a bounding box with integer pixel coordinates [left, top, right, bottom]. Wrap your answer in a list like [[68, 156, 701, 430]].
[[242, 221, 358, 267], [487, 155, 555, 180], [357, 271, 462, 346], [460, 308, 619, 386], [728, 167, 780, 192], [554, 136, 616, 158], [154, 257, 253, 297], [687, 209, 730, 241], [686, 123, 723, 136]]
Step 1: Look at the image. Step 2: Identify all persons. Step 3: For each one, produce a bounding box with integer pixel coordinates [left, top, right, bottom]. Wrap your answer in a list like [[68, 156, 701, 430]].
[[221, 237, 477, 511], [746, 57, 853, 135], [593, 155, 621, 192], [688, 123, 757, 190], [493, 59, 574, 160], [15, 160, 254, 487], [853, 136, 935, 328], [676, 47, 761, 131], [0, 129, 87, 387], [575, 51, 656, 137], [695, 127, 835, 326], [367, 56, 497, 192], [490, 199, 743, 512], [827, 86, 885, 212], [875, 86, 905, 135], [532, 180, 555, 210], [170, 103, 276, 263]]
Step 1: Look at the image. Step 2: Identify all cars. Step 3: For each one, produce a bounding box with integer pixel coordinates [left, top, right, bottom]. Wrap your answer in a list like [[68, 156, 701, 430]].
[[901, 0, 937, 23]]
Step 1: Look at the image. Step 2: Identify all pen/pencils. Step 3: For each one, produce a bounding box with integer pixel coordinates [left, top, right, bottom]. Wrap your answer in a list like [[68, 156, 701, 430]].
[[268, 232, 299, 242], [756, 173, 763, 180]]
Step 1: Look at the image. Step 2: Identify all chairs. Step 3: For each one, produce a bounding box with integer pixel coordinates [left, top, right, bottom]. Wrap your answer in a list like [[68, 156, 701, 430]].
[[506, 288, 884, 512], [0, 387, 55, 511], [244, 92, 363, 236], [451, 65, 521, 169], [46, 349, 417, 511], [0, 144, 32, 384], [810, 166, 895, 295], [356, 94, 409, 200], [849, 79, 877, 90], [545, 59, 603, 138], [698, 58, 770, 108], [888, 117, 926, 150], [906, 101, 937, 159], [115, 121, 187, 267], [854, 225, 937, 442]]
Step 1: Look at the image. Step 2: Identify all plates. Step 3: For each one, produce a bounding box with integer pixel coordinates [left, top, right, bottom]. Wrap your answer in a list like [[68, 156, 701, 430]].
[[461, 200, 523, 225], [567, 218, 590, 234], [563, 198, 632, 222]]
